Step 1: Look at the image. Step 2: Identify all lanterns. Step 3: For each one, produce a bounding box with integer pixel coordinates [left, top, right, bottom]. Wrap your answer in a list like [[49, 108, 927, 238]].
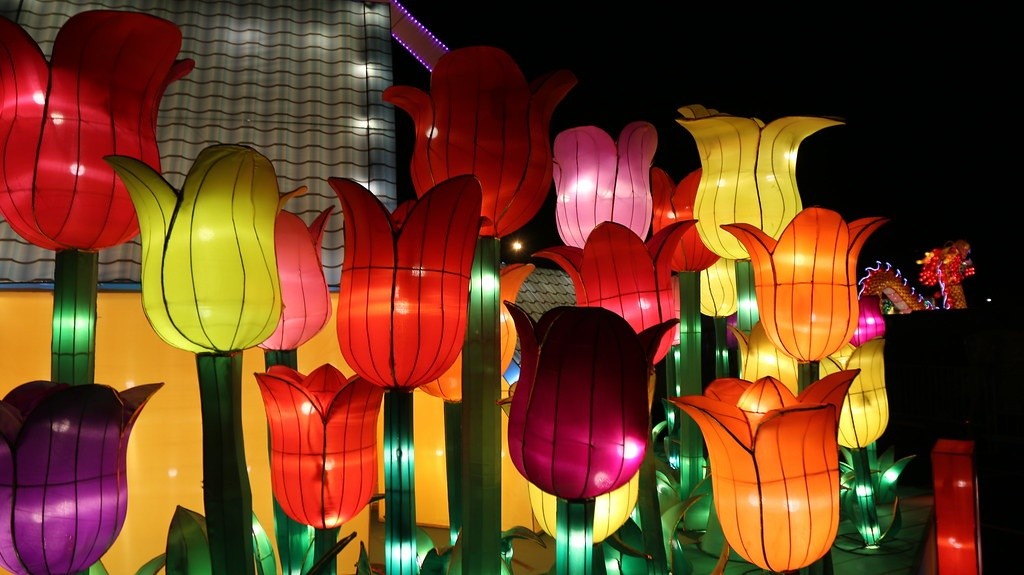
[[0, 9, 975, 575]]
